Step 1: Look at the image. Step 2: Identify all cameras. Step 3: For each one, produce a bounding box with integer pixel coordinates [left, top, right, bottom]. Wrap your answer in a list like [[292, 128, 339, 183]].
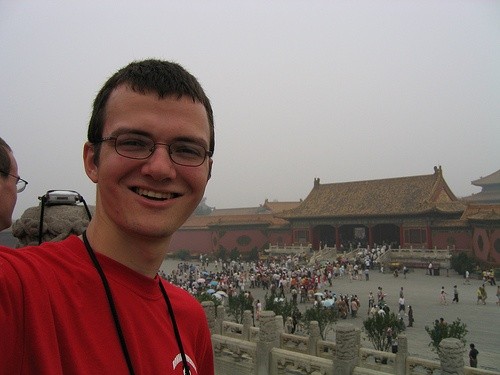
[[44, 191, 79, 205]]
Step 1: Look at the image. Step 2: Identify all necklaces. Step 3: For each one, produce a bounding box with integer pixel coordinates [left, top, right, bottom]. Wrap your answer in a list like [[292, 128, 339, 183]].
[[81, 230, 196, 375]]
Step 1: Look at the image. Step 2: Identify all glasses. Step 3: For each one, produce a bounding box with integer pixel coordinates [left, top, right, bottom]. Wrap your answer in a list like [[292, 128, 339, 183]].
[[93, 132, 213, 168], [0, 169, 28, 194]]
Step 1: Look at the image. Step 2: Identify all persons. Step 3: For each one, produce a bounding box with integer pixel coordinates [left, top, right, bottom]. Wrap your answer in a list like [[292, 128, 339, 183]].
[[159, 239, 500, 368], [0, 137, 20, 233], [0, 60, 214, 375]]
